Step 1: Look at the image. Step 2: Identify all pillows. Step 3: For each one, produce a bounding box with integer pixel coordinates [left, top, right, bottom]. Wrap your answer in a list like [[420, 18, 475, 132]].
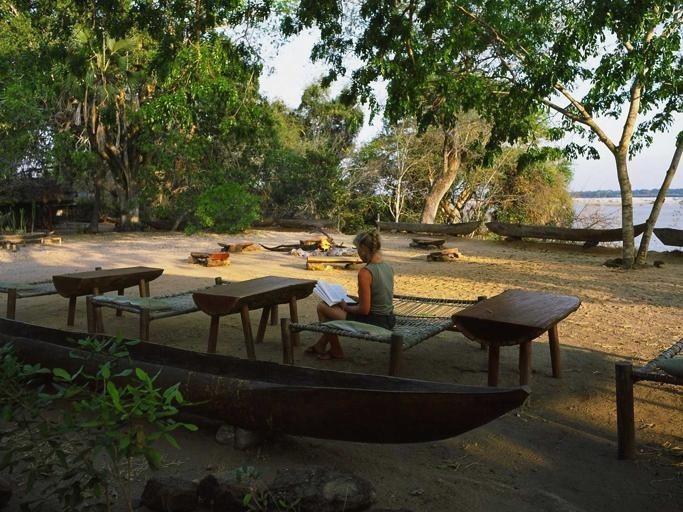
[[322, 319, 392, 339], [94, 295, 174, 311], [655, 358, 683, 378]]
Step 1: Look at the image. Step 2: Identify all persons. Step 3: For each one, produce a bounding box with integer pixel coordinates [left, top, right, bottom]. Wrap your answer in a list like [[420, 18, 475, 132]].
[[304, 228, 395, 360]]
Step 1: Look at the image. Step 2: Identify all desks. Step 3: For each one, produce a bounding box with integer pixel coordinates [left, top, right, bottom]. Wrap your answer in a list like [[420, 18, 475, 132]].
[[50, 266, 168, 332], [451, 288, 583, 385], [192, 276, 320, 361]]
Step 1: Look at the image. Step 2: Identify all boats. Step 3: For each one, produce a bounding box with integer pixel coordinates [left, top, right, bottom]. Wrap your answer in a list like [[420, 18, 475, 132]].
[[277, 216, 336, 228], [0, 318, 531, 442], [652, 228, 683, 247], [375, 219, 480, 236], [483, 221, 647, 242]]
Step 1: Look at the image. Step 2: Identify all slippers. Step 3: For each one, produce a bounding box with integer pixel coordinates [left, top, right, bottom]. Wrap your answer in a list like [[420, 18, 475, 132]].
[[305, 346, 325, 354], [319, 352, 344, 360]]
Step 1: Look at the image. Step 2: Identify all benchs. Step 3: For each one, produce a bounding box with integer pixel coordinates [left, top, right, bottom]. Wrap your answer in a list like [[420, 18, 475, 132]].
[[614, 332, 682, 468], [0, 280, 62, 321], [85, 277, 278, 340], [279, 290, 489, 377]]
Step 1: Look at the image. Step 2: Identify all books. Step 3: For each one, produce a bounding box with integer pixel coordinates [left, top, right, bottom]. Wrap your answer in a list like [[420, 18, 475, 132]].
[[312, 280, 357, 307]]
